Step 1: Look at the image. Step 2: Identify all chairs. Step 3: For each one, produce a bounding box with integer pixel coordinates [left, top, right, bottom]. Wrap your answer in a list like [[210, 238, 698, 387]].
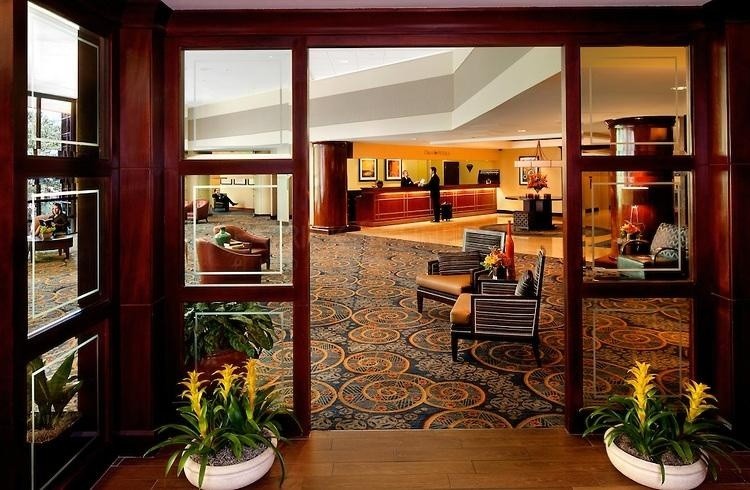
[[415, 227, 507, 313], [185, 193, 270, 284], [617, 223, 689, 280], [450, 249, 545, 367]]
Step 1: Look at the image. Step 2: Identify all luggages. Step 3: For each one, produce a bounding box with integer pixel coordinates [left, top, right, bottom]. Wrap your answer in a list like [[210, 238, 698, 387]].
[[440, 201, 453, 222]]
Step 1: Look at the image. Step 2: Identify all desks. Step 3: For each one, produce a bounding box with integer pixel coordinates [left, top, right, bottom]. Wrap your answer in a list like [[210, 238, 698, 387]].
[[478, 274, 506, 294], [505, 196, 562, 231], [27, 234, 73, 259]]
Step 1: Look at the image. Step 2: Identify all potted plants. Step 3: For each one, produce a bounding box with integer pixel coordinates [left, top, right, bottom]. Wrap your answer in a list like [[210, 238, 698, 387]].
[[140, 359, 304, 490], [579, 361, 742, 490], [40, 225, 56, 239], [183, 301, 279, 394], [27, 350, 83, 450]]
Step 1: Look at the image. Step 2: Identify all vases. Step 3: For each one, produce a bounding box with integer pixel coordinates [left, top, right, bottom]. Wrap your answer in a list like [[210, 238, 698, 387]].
[[491, 265, 505, 279]]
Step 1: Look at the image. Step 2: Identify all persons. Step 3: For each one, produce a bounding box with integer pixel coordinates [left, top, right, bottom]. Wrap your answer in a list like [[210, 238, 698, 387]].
[[401, 170, 413, 187], [418, 166, 441, 222], [212, 188, 237, 212], [35, 203, 68, 238]]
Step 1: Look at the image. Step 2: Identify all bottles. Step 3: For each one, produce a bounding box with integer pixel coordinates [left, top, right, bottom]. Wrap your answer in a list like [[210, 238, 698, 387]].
[[216, 226, 231, 246]]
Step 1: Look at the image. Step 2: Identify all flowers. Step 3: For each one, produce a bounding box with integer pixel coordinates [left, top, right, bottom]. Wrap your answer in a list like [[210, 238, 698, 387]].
[[480, 245, 511, 270]]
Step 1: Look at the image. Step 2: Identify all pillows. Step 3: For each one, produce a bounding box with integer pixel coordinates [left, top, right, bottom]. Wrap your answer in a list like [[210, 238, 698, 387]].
[[515, 270, 534, 297], [438, 252, 482, 275]]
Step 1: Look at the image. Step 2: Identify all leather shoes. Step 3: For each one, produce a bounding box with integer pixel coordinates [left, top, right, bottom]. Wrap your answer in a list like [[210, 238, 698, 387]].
[[232, 203, 238, 206]]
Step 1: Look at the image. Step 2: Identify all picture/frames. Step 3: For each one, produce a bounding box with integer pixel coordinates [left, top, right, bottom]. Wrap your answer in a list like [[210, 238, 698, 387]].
[[220, 178, 254, 185], [519, 155, 539, 185], [385, 159, 402, 181], [359, 158, 378, 182]]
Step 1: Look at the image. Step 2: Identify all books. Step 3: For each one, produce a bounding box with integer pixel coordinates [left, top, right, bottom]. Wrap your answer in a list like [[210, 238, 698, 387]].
[[38, 219, 52, 226]]
